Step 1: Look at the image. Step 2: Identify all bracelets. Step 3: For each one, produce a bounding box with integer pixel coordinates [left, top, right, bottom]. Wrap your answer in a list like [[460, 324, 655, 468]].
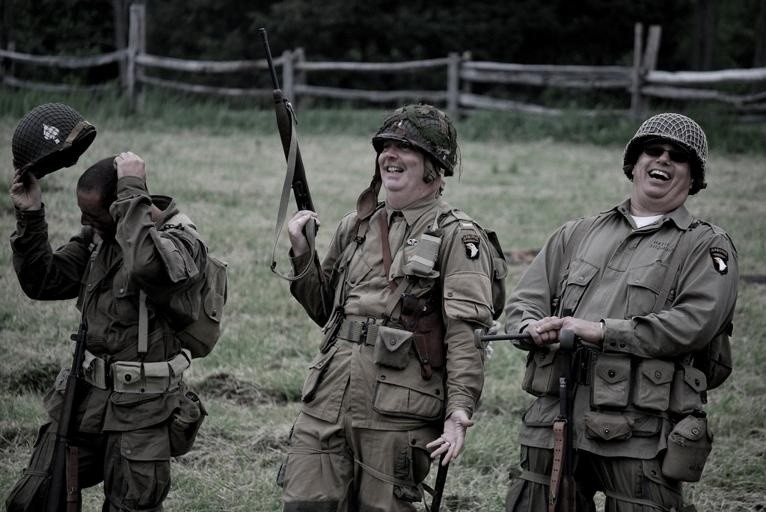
[[600, 321, 605, 340]]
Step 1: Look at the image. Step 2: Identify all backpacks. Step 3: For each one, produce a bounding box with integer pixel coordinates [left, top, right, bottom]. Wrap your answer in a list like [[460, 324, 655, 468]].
[[161, 212, 229, 359]]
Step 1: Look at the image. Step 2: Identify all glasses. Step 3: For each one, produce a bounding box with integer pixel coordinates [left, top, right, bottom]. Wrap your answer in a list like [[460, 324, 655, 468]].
[[641, 145, 696, 163]]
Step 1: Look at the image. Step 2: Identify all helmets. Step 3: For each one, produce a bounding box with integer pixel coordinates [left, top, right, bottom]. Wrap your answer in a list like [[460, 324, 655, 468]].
[[12, 101, 98, 185], [623, 111, 709, 197], [371, 103, 458, 177]]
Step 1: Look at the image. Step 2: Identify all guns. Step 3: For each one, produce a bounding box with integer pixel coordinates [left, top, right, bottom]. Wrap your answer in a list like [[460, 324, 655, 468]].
[[552, 328, 579, 511], [261, 27, 318, 235], [44, 327, 84, 511]]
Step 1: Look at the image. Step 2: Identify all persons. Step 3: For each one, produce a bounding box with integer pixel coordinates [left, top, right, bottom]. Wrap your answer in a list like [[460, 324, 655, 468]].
[[276, 104, 508, 512], [5, 151, 227, 512], [505, 113, 739, 512]]
